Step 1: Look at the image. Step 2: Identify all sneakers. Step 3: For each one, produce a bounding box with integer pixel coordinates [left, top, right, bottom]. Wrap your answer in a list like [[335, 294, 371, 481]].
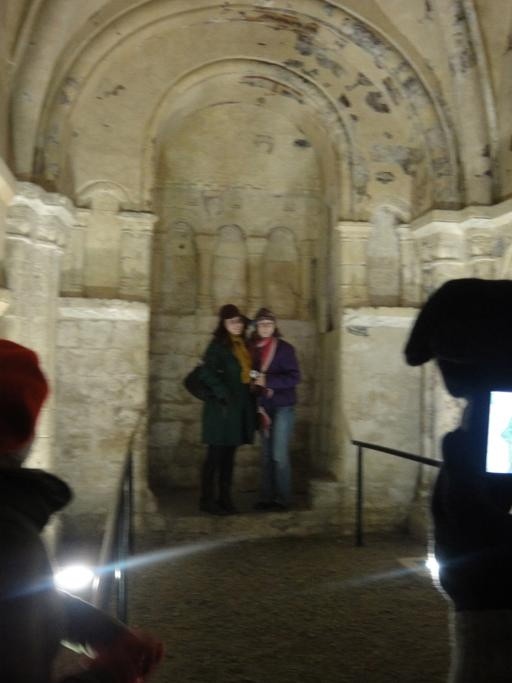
[[200, 497, 237, 515]]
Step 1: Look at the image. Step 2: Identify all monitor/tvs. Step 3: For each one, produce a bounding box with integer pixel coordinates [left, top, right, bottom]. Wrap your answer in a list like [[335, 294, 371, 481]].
[[478, 381, 512, 478]]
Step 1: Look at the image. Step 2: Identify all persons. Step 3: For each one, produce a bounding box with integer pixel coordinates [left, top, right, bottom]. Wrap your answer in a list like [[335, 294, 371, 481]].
[[193, 300, 264, 520], [2, 338, 166, 683], [403, 277, 512, 681], [236, 305, 304, 517]]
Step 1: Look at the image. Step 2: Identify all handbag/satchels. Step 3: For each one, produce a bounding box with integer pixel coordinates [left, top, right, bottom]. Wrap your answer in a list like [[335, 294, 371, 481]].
[[182, 364, 214, 405]]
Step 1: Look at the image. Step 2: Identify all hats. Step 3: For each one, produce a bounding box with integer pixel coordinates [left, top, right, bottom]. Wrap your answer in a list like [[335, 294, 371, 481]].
[[254, 306, 276, 323]]
